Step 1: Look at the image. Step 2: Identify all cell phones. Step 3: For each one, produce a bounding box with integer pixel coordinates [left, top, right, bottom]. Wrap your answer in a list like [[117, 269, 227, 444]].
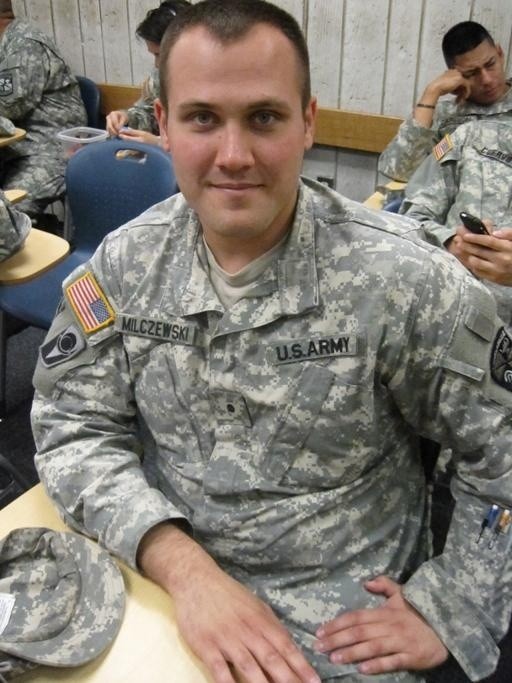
[[460, 212, 489, 235]]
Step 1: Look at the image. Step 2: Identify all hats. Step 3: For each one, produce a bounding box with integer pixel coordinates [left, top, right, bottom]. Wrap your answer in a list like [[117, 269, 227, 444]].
[[1, 527, 127, 681]]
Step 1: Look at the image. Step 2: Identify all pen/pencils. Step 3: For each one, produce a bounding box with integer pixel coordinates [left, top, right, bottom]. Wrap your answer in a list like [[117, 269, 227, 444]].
[[476, 504, 511, 550]]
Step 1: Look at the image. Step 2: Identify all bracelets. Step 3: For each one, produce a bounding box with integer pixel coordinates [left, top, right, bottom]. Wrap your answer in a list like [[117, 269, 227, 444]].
[[415, 103, 438, 110]]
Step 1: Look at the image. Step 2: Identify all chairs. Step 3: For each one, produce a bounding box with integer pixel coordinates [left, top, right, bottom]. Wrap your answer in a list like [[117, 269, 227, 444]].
[[0, 128, 27, 146], [0, 139, 177, 419], [75, 75, 101, 128]]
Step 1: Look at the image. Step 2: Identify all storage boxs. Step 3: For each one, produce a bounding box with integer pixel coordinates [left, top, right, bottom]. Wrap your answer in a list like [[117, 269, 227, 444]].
[[58, 127, 109, 158]]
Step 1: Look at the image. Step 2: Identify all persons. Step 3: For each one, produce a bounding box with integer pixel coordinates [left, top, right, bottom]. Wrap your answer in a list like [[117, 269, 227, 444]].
[[1, 187, 36, 340], [375, 20, 512, 183], [398, 120, 512, 334], [1, 0, 89, 227], [27, 1, 510, 683], [104, 0, 194, 159]]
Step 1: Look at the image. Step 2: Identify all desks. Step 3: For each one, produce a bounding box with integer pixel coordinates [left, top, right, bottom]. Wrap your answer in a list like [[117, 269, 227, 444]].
[[0, 189, 68, 285], [362, 181, 406, 211], [0, 479, 214, 682]]
